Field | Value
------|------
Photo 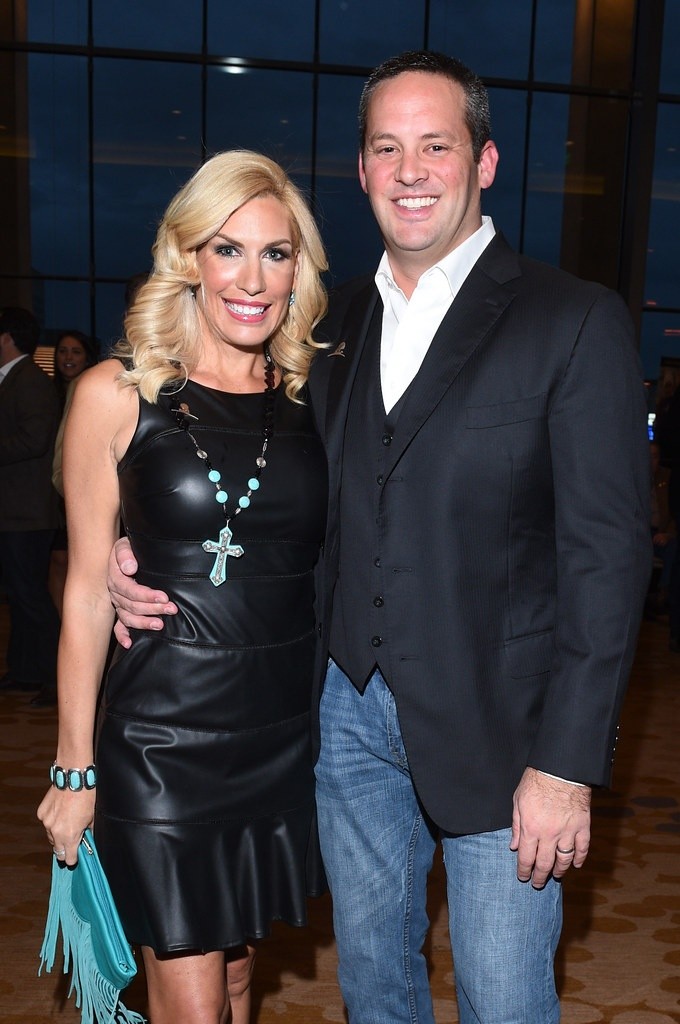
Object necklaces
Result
[167,338,275,588]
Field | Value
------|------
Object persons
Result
[107,50,654,1024]
[36,149,332,1024]
[0,306,99,707]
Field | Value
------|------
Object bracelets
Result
[49,760,97,792]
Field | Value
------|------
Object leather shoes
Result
[0,664,56,709]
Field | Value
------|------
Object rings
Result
[557,845,574,853]
